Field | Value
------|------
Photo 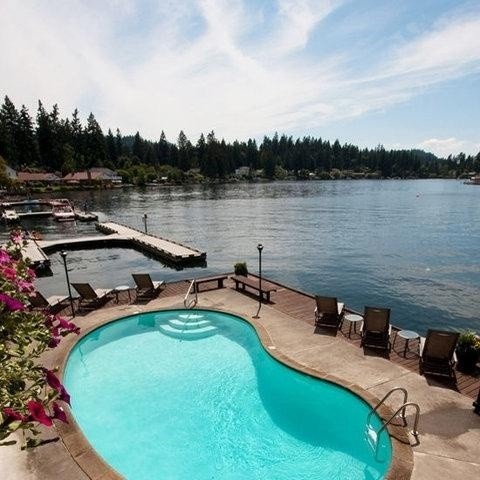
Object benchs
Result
[183,272,277,305]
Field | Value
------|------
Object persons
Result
[83,199,93,215]
[37,229,42,240]
[69,198,77,211]
[23,228,30,240]
[31,228,37,240]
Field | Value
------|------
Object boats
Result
[51,199,75,222]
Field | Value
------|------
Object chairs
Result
[312,294,461,382]
[25,273,165,309]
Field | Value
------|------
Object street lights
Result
[59,251,75,317]
[257,243,264,308]
